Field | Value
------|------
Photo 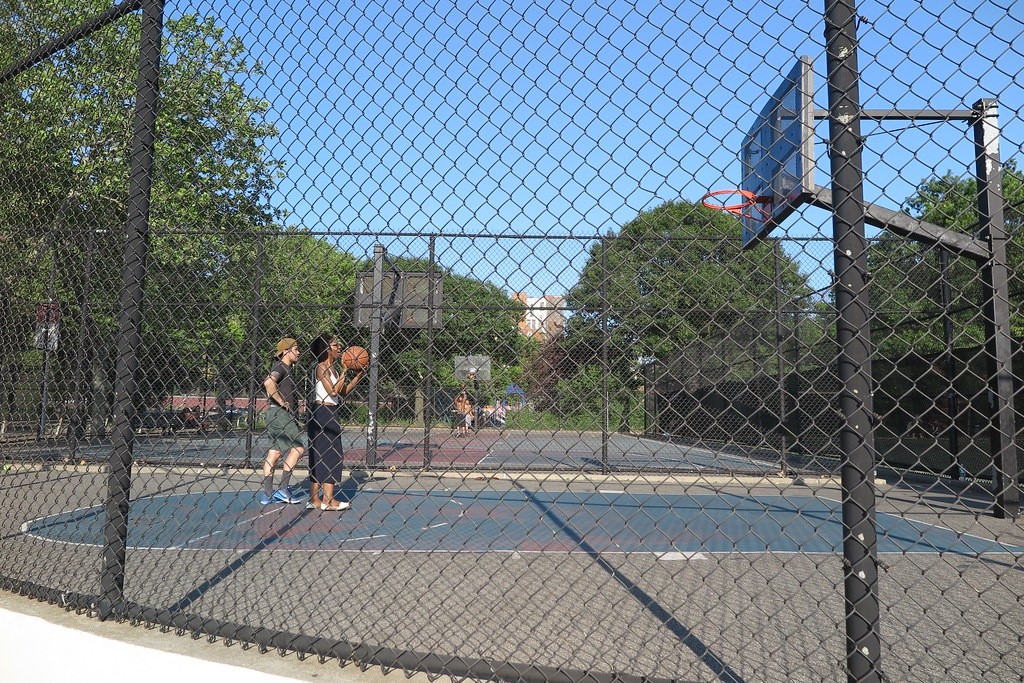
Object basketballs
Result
[344,346,368,370]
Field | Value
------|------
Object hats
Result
[274,338,298,357]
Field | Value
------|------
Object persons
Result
[306,333,369,510]
[454,394,471,437]
[178,406,207,432]
[259,338,304,504]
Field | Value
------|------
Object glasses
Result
[330,343,338,348]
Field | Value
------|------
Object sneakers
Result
[271,487,300,503]
[260,492,284,504]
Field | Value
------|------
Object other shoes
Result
[306,502,321,508]
[462,431,465,438]
[455,432,460,438]
[320,502,351,511]
